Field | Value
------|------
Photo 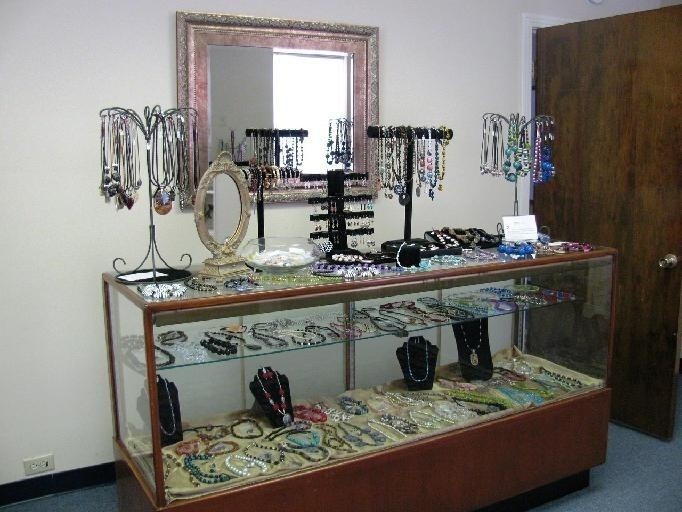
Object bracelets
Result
[244,167,301,193]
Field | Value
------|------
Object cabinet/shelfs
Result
[101,236,619,511]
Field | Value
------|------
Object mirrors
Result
[193,150,251,255]
[175,11,380,207]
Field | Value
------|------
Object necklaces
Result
[119,228,595,490]
[247,125,305,167]
[322,119,450,205]
[481,112,557,186]
[94,102,200,214]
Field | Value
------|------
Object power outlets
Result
[22,453,55,475]
